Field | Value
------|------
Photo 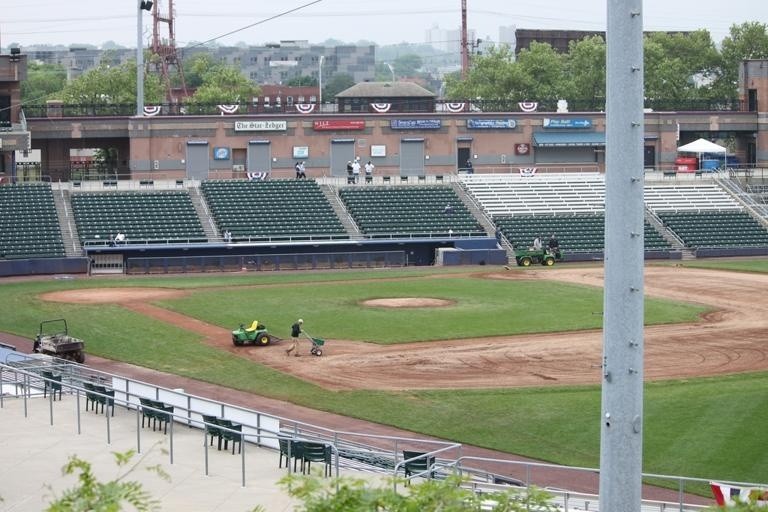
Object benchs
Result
[459,172,745,218]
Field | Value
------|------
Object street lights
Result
[439,80,448,110]
[381,60,395,82]
[317,54,324,110]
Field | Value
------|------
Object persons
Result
[495,226,501,244]
[533,234,542,249]
[444,204,453,213]
[549,234,558,248]
[108,233,114,247]
[223,229,232,242]
[115,230,126,244]
[364,161,375,182]
[300,161,306,178]
[285,319,304,357]
[352,160,361,183]
[295,162,300,178]
[465,159,474,174]
[346,161,355,184]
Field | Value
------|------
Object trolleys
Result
[299,329,325,356]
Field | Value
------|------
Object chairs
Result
[490,207,768,253]
[274,433,302,473]
[214,416,243,455]
[201,412,220,450]
[150,399,174,433]
[1,174,488,262]
[40,368,64,402]
[82,380,99,414]
[93,384,116,418]
[300,440,333,478]
[402,448,436,487]
[139,397,157,431]
